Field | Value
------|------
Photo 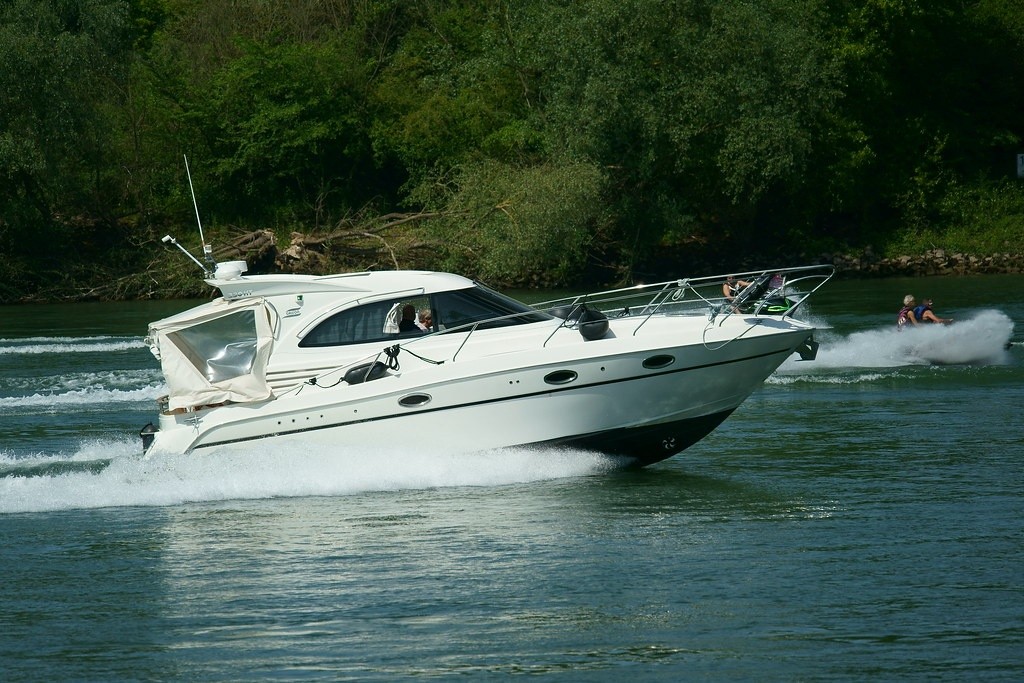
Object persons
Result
[399,305,432,334]
[723,276,750,314]
[898,294,952,334]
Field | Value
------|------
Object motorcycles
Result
[716,269,799,318]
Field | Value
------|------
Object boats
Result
[135,150,838,473]
